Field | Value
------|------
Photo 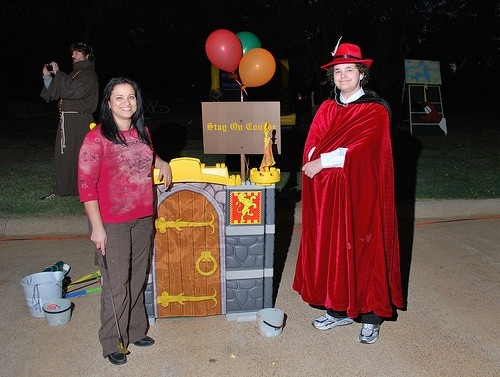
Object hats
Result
[320,35,374,71]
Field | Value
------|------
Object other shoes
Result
[41,192,59,200]
[108,351,127,365]
[133,336,155,346]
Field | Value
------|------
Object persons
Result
[41,43,97,199]
[78,78,172,365]
[292,43,403,344]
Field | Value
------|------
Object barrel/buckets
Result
[20,271,64,318]
[44,299,72,326]
[256,308,284,338]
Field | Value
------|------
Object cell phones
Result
[46,65,52,72]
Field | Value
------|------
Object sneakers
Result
[358,322,380,344]
[311,311,354,331]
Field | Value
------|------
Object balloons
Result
[205,29,242,73]
[236,32,261,55]
[239,48,276,87]
